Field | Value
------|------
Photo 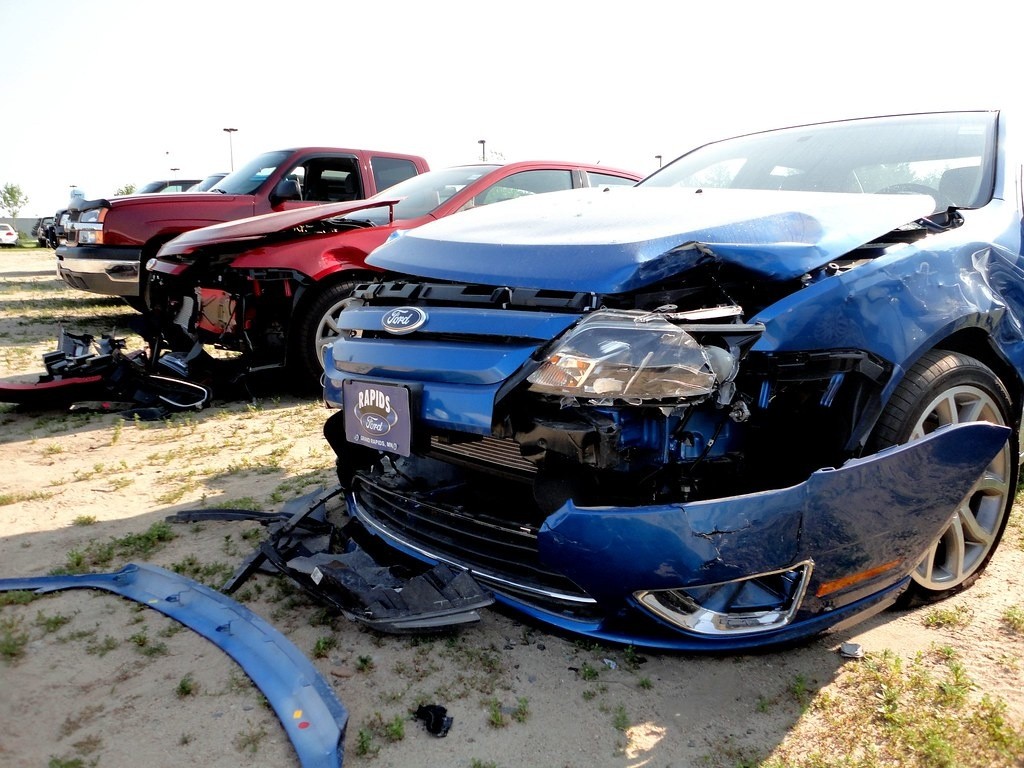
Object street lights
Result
[224,128,238,170]
[655,155,661,168]
[478,140,485,161]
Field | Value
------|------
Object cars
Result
[318,108,1024,655]
[1,224,19,248]
[38,217,54,247]
[186,173,457,205]
[145,163,646,399]
[48,180,203,250]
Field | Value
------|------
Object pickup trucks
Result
[54,147,440,313]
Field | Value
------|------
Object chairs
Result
[780,172,837,192]
[938,165,983,208]
[340,173,361,199]
